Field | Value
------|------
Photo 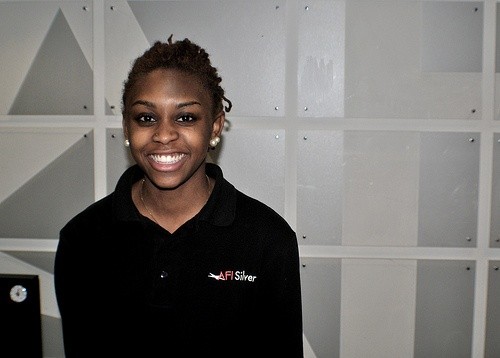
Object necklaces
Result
[139,175,212,233]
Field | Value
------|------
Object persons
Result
[53,33,304,358]
